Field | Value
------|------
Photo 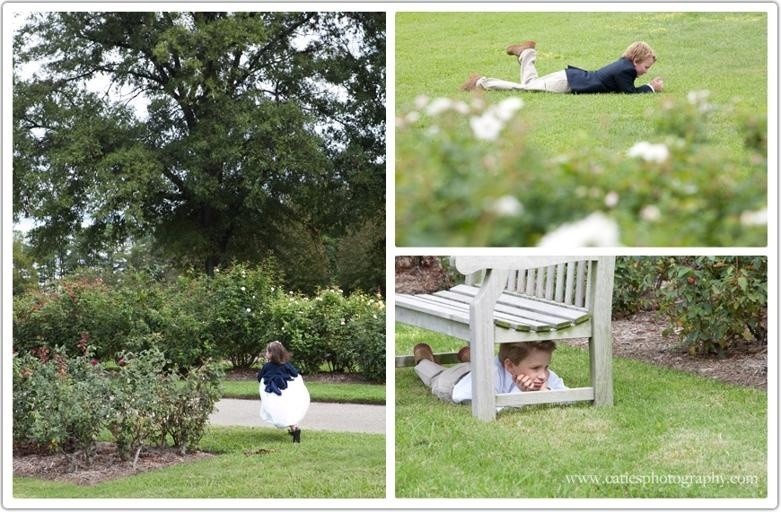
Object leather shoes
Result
[288,428,301,443]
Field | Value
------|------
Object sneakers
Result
[457,344,470,362]
[505,41,536,57]
[459,71,481,93]
[413,342,440,366]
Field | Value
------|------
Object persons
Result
[257,341,311,442]
[459,40,665,95]
[413,341,578,414]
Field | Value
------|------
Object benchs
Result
[395,254,617,423]
